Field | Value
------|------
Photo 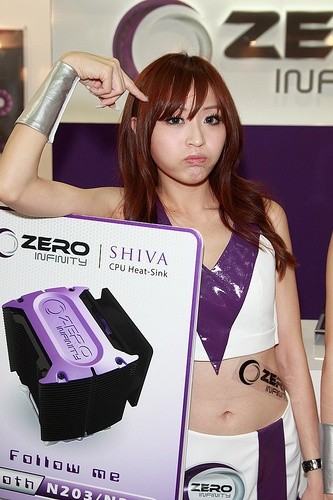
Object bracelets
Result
[302,457,323,473]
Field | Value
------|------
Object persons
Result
[322,232,333,500]
[0,50,325,500]
